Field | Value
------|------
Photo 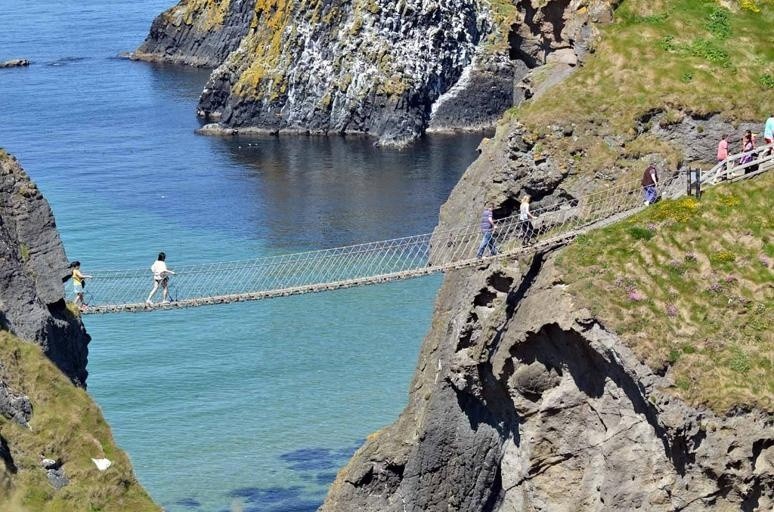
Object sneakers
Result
[644,202,649,208]
[163,300,170,304]
[147,300,153,305]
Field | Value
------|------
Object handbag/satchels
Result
[81,280,85,288]
[163,276,169,282]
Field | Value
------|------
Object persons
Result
[740,129,758,174]
[475,202,501,260]
[641,161,659,207]
[146,252,176,305]
[68,261,92,308]
[763,114,774,159]
[518,194,538,248]
[717,134,732,181]
